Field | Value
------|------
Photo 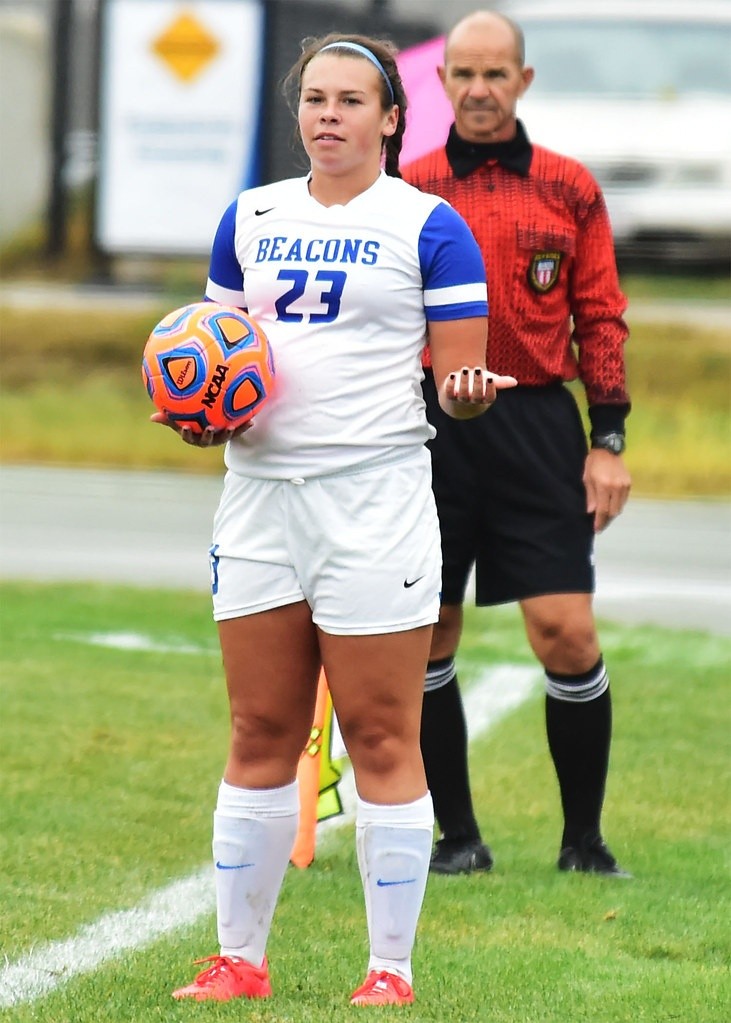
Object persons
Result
[137,34,522,1012]
[371,7,648,890]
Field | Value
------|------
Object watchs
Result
[591,431,628,458]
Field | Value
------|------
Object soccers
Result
[138,298,279,438]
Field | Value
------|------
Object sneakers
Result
[429,838,494,876]
[558,840,632,881]
[350,967,414,1008]
[171,953,271,1002]
